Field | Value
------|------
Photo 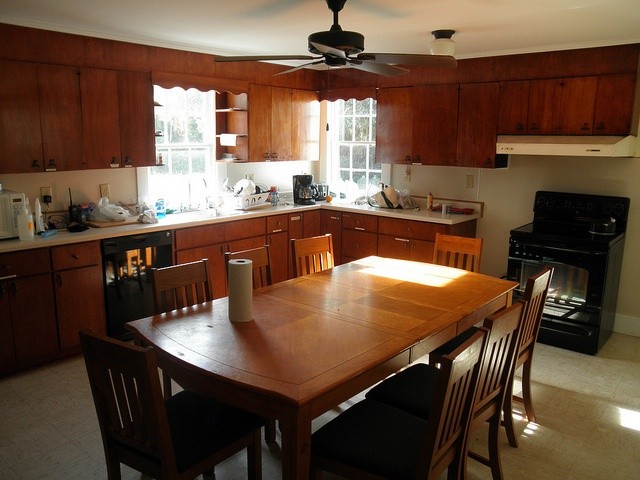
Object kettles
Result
[588,214,617,236]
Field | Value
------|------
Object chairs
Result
[291,233,335,278]
[430,231,483,272]
[150,258,215,399]
[224,244,276,445]
[310,327,491,480]
[366,300,527,480]
[429,265,554,448]
[78,328,267,479]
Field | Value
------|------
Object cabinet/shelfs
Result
[175,218,266,308]
[342,212,378,264]
[378,218,477,271]
[216,84,320,163]
[457,82,499,168]
[0,240,106,377]
[375,84,459,166]
[497,79,562,135]
[80,67,165,170]
[561,73,636,136]
[267,214,289,283]
[0,61,84,174]
[321,210,342,267]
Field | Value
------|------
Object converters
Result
[44,195,52,204]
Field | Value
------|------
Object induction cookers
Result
[512,221,623,242]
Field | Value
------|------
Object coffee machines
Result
[293,175,318,205]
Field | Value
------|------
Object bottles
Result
[270,187,278,207]
[426,192,434,211]
[18,205,34,240]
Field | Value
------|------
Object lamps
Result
[429,30,456,56]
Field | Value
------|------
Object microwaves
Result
[0,189,25,240]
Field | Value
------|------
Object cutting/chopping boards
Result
[81,216,143,227]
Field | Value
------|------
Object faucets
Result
[188,177,210,209]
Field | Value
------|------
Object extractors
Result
[495,135,640,159]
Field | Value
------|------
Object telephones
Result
[67,186,82,223]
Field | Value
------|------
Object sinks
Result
[186,207,248,220]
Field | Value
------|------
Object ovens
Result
[505,238,625,355]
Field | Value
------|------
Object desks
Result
[122,254,519,480]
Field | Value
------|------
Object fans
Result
[213,0,457,77]
